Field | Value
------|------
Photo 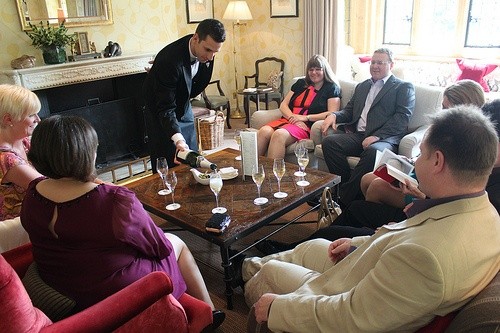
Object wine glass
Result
[163,169,181,211]
[234,128,242,161]
[272,158,289,198]
[209,170,227,213]
[251,163,268,206]
[293,140,310,186]
[156,156,172,195]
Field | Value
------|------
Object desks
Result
[237,87,275,128]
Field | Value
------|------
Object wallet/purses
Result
[205,213,231,235]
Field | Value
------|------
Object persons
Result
[20,114,224,329]
[222,103,499,333]
[0,83,117,219]
[256,79,500,255]
[142,18,228,174]
[321,48,416,206]
[256,54,345,160]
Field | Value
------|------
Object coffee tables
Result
[124,148,341,311]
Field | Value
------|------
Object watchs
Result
[306,114,309,122]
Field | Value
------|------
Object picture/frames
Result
[270,0,298,18]
[185,0,215,24]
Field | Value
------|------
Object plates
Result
[218,172,239,180]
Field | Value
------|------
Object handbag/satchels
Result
[318,187,342,229]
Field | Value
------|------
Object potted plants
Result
[23,19,76,65]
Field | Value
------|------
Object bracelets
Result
[374,135,379,141]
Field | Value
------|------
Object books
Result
[373,148,419,189]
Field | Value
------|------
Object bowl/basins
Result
[189,168,211,186]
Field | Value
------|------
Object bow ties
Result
[190,60,199,65]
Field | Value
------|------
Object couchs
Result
[0,241,213,333]
[250,77,447,175]
[247,270,500,333]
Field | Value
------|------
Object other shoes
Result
[227,249,245,291]
[254,239,289,256]
[199,310,225,333]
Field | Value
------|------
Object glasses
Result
[369,61,391,65]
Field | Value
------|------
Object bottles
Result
[176,148,218,171]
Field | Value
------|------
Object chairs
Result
[190,80,232,129]
[245,56,284,124]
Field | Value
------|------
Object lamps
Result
[222,1,253,118]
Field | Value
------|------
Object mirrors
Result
[15,0,114,32]
